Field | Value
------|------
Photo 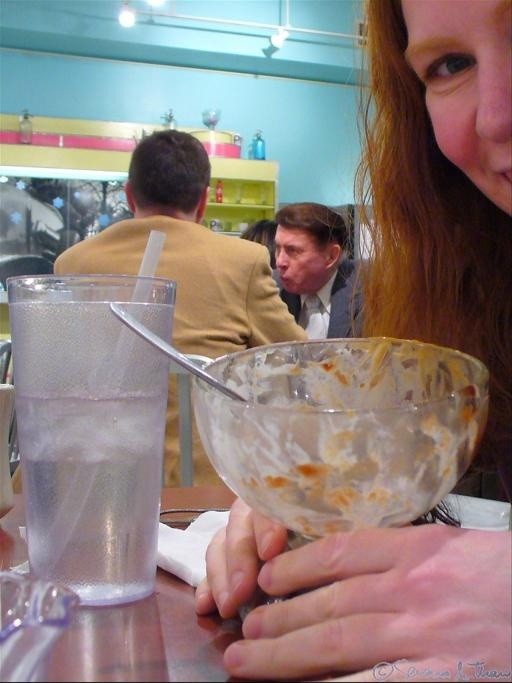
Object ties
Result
[304,295,326,339]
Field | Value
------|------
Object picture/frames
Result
[1,163,142,306]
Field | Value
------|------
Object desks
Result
[2,479,367,681]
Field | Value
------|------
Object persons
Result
[196,0,511,682]
[52,130,311,486]
[23,175,95,245]
[239,221,279,269]
[271,203,376,339]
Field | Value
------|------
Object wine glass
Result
[202,108,222,131]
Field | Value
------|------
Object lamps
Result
[117,0,293,49]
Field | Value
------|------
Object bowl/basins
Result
[192,338,492,619]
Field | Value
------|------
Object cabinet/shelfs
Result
[199,156,281,238]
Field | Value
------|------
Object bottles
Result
[18,110,36,144]
[251,128,268,160]
[215,179,223,202]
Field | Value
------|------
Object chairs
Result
[0,336,21,476]
[169,352,215,489]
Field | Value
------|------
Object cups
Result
[1,272,180,607]
[210,219,223,231]
[238,223,249,232]
